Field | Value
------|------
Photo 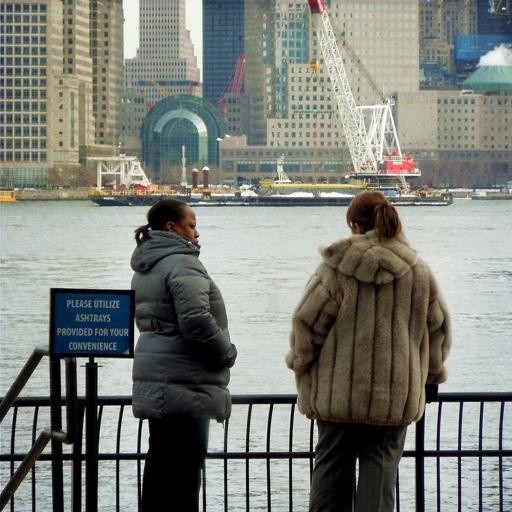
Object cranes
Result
[307,1,423,190]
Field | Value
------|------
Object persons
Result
[284,188,452,511]
[130,196,239,512]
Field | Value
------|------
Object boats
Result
[89,192,453,207]
